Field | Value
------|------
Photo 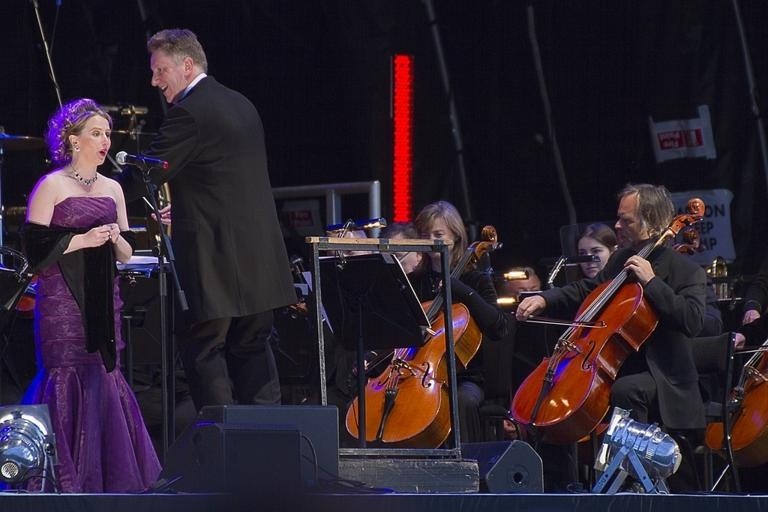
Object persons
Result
[512,180,709,484]
[741,258,766,326]
[328,203,628,450]
[128,28,293,411]
[726,318,768,352]
[25,96,166,494]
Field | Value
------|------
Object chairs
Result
[589,272,745,494]
[447,311,523,463]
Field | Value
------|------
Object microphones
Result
[116,151,169,170]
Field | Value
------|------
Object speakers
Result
[156,405,340,495]
[460,440,546,493]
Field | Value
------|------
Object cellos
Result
[345,225,499,448]
[707,339,768,466]
[511,198,705,445]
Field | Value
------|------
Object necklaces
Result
[71,166,98,186]
